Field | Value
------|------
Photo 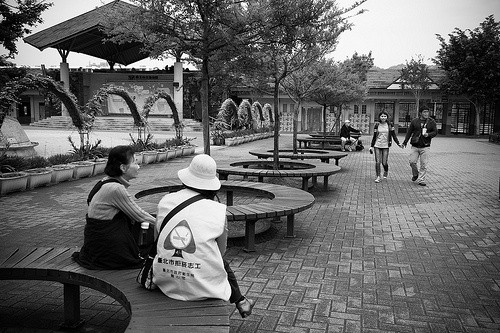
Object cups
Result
[370,149,373,154]
[422,128,427,136]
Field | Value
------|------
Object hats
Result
[177,154,221,190]
[345,120,350,123]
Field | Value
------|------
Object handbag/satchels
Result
[136,252,158,289]
[411,137,419,143]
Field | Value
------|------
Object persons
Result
[340,120,363,152]
[369,111,404,183]
[401,105,437,186]
[84,146,157,270]
[152,154,256,318]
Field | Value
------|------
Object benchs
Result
[0,131,361,333]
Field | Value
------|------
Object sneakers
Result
[375,176,382,183]
[236,297,255,318]
[383,175,388,179]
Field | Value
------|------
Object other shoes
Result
[412,174,418,181]
[419,180,426,186]
[348,147,352,151]
[341,148,345,151]
